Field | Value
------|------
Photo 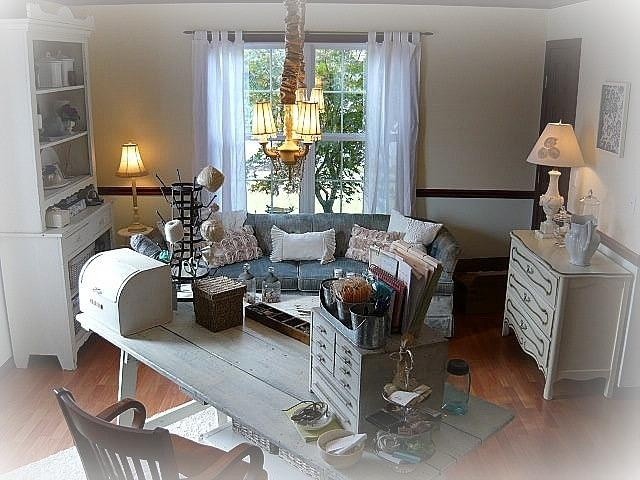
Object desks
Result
[75,302,516,479]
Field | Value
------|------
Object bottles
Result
[239,264,257,304]
[553,205,570,248]
[579,189,600,223]
[443,358,470,416]
[261,267,281,303]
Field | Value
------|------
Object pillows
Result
[215,225,262,266]
[269,225,336,264]
[387,210,444,253]
[344,224,404,265]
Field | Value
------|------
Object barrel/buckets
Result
[335,294,372,328]
[349,305,386,349]
[322,278,341,315]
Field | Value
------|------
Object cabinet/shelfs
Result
[0,2,100,233]
[308,306,450,440]
[501,228,634,400]
[0,197,113,372]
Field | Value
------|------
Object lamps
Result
[526,119,585,241]
[252,1,325,184]
[116,139,150,232]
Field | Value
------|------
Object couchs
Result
[208,213,463,338]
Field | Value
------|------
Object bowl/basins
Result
[317,429,365,467]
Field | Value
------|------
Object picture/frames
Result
[594,83,630,158]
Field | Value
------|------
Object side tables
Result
[116,226,154,249]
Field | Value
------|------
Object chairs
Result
[53,386,268,479]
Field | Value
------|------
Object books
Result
[364,240,441,335]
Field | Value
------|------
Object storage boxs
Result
[192,276,247,333]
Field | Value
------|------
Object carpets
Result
[0,398,313,479]
